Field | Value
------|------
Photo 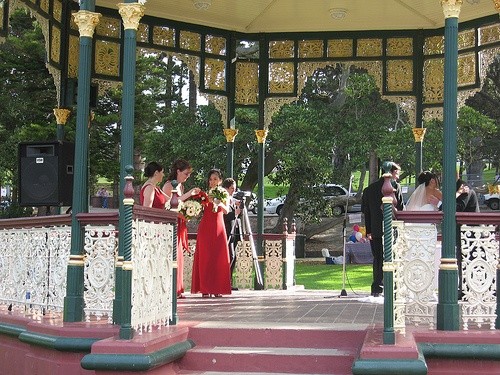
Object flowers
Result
[165,184,228,222]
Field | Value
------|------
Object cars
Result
[266,183,351,217]
[482,193,500,210]
[243,191,259,214]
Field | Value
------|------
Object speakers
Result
[17,141,75,207]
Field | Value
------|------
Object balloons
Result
[348,224,367,242]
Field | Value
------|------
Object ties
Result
[230,198,233,205]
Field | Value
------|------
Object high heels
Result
[202,294,209,297]
[210,293,222,297]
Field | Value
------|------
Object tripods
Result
[227,200,264,289]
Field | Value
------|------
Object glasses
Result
[182,171,190,177]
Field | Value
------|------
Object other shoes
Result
[231,286,238,290]
[177,294,185,299]
[371,286,384,297]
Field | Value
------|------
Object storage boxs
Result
[346,243,374,264]
[325,257,335,264]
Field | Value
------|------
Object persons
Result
[426,171,487,301]
[403,170,469,305]
[98,186,109,209]
[222,178,240,290]
[162,160,201,298]
[191,169,232,298]
[361,161,406,297]
[140,161,170,210]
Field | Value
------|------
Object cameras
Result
[233,191,256,202]
